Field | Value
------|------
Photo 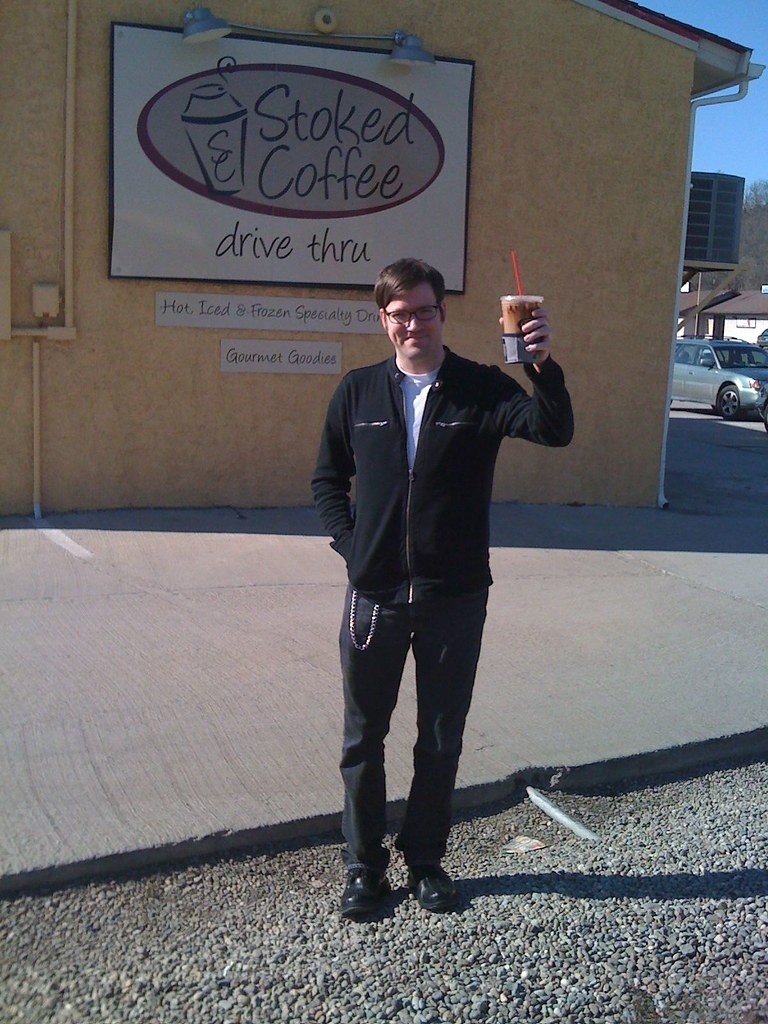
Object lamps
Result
[178,3,438,68]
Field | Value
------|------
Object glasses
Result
[383,299,441,325]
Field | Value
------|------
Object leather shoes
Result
[340,869,389,916]
[407,866,458,911]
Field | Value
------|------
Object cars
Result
[757,328,768,348]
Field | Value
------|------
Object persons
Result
[309,260,575,919]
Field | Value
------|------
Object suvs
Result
[668,333,768,420]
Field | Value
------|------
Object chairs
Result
[676,351,689,363]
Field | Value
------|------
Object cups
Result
[500,294,545,364]
